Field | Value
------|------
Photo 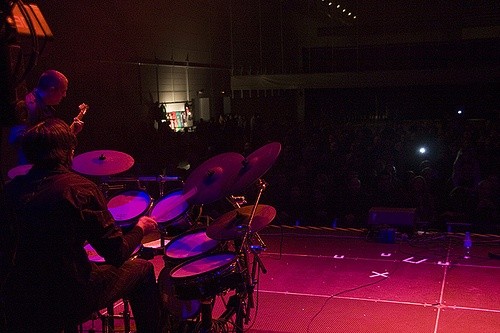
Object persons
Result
[133,112,500,234]
[0,120,172,333]
[13,70,85,166]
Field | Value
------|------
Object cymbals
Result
[205,204,277,241]
[182,152,244,204]
[71,150,135,175]
[221,142,281,194]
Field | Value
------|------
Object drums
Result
[164,226,228,271]
[106,188,153,232]
[169,250,237,300]
[147,188,203,240]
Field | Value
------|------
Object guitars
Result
[70,103,88,134]
